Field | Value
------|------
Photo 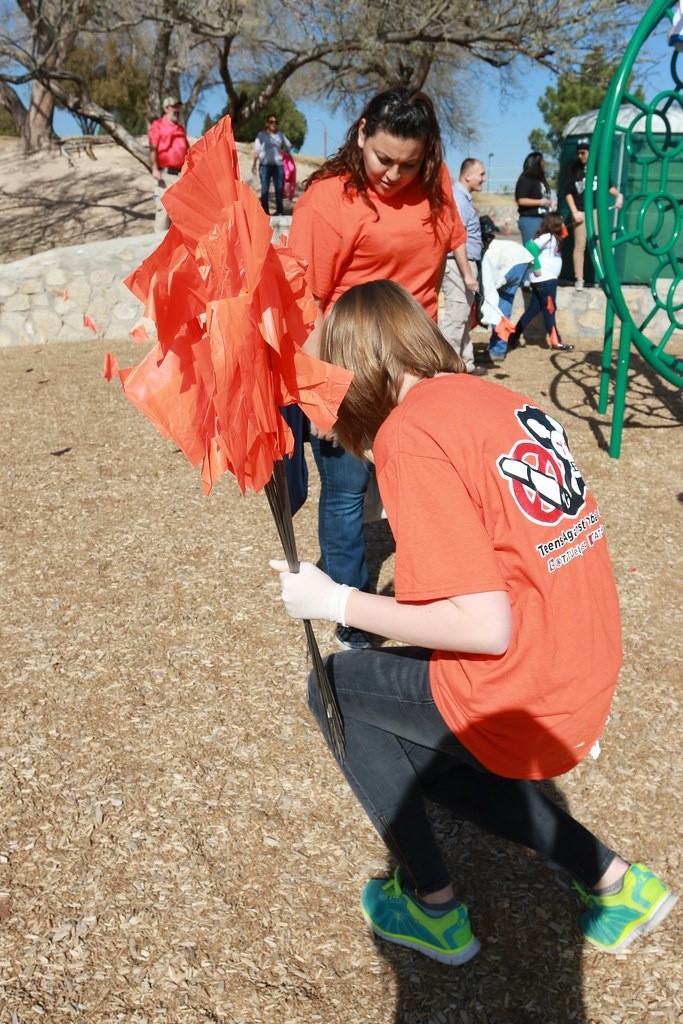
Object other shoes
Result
[553,342,574,353]
[334,623,372,652]
[575,280,585,290]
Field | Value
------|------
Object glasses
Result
[269,120,278,124]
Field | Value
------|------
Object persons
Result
[474,214,574,363]
[441,158,487,375]
[564,141,623,292]
[514,151,551,291]
[269,279,680,968]
[251,114,292,215]
[286,89,466,653]
[148,96,190,232]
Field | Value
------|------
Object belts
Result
[160,167,183,176]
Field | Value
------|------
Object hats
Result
[162,96,183,108]
[576,135,591,150]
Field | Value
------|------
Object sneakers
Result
[570,862,680,954]
[360,864,482,967]
